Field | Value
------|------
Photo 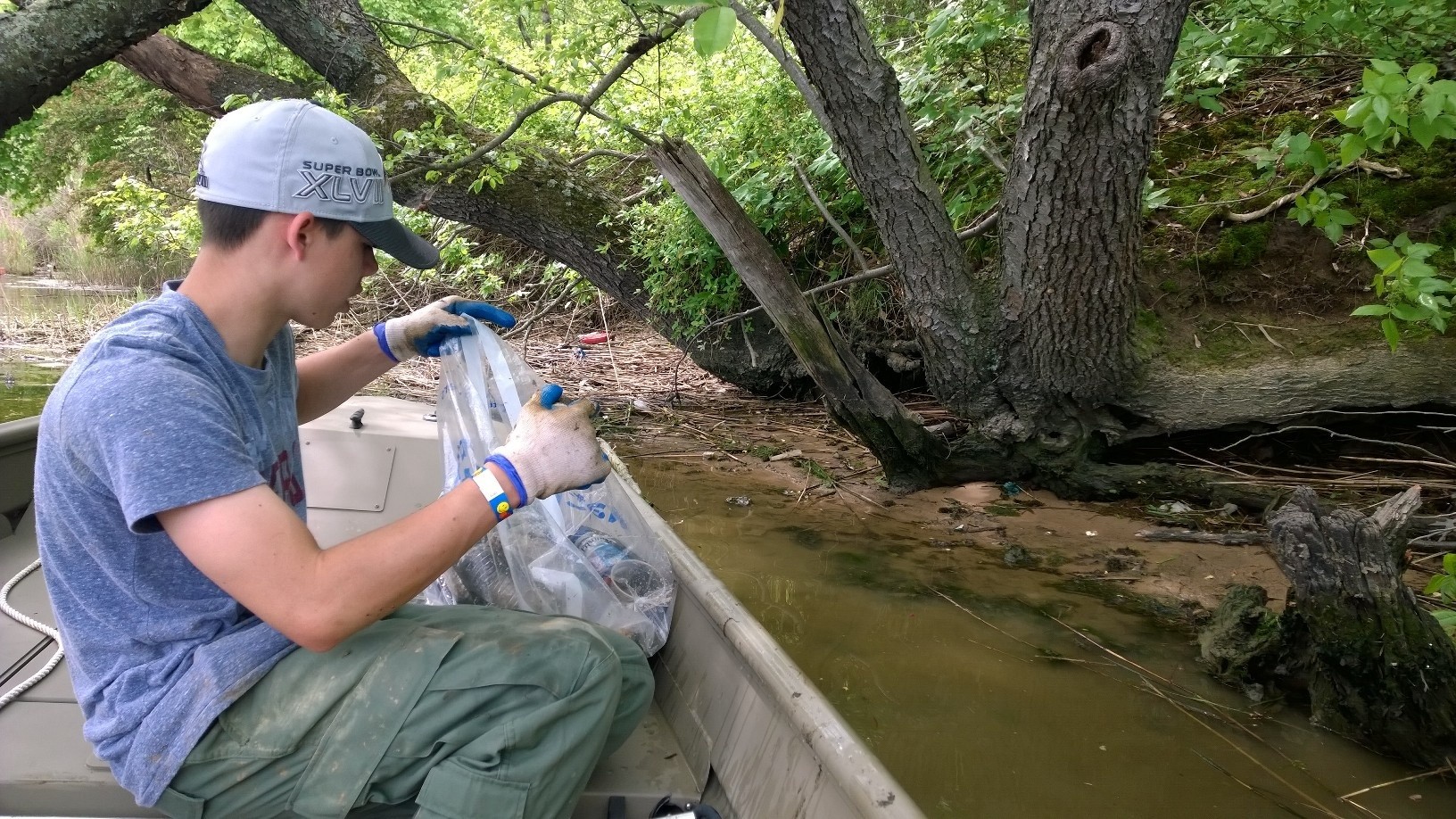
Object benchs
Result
[0,701,713,817]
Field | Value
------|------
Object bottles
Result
[565,526,652,601]
[454,544,526,611]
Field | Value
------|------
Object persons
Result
[33,99,653,818]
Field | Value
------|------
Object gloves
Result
[373,295,516,364]
[483,384,612,510]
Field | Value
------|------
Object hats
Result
[192,97,440,270]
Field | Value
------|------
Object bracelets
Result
[468,468,515,522]
